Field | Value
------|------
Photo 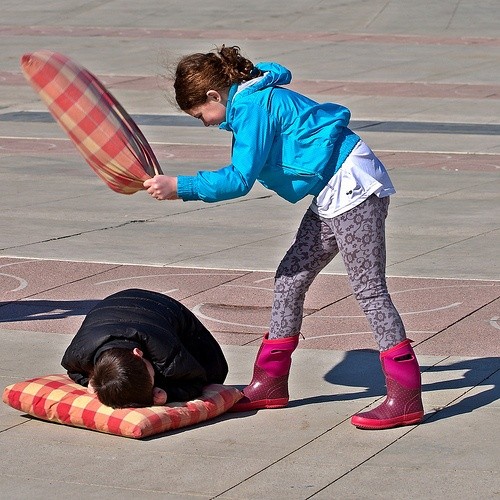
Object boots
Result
[217,332,298,413]
[350,338,426,432]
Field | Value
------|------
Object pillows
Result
[2,375,244,438]
[21,50,163,195]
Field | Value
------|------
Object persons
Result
[142,43,426,430]
[61,289,233,408]
